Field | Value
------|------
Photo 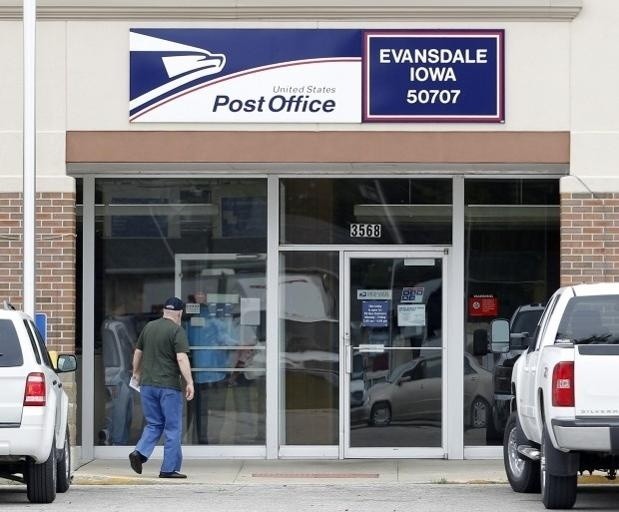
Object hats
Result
[163,297,184,310]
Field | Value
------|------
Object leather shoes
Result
[129,450,142,474]
[159,472,187,478]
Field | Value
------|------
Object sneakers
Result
[98,429,119,446]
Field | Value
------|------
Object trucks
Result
[140,274,367,425]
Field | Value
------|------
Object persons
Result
[98,294,133,446]
[185,288,266,444]
[128,298,196,478]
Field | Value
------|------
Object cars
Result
[0,311,77,503]
[364,302,550,445]
[103,321,136,446]
[503,282,619,508]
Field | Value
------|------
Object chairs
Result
[573,309,604,340]
